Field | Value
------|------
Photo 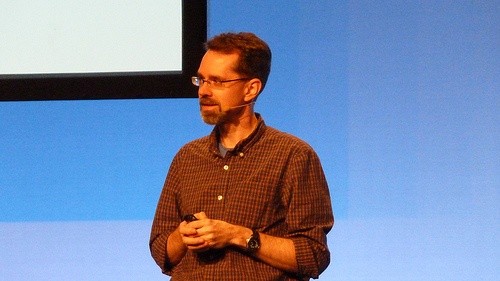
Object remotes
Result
[184,214,198,224]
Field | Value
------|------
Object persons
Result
[148,31,334,281]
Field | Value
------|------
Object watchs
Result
[245,228,261,255]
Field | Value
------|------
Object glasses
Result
[191,76,252,88]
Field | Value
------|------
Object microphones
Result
[222,102,255,112]
